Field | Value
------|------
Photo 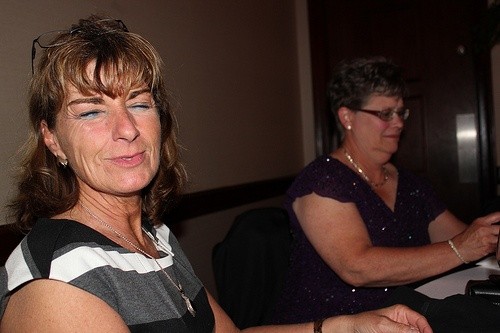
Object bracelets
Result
[312,315,325,333]
[443,237,471,267]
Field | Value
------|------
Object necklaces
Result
[74,194,198,319]
[338,142,389,187]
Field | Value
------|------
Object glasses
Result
[349,107,409,121]
[31,18,128,73]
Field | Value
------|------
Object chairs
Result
[212,206,289,330]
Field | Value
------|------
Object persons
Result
[286,58,499,313]
[0,13,440,333]
